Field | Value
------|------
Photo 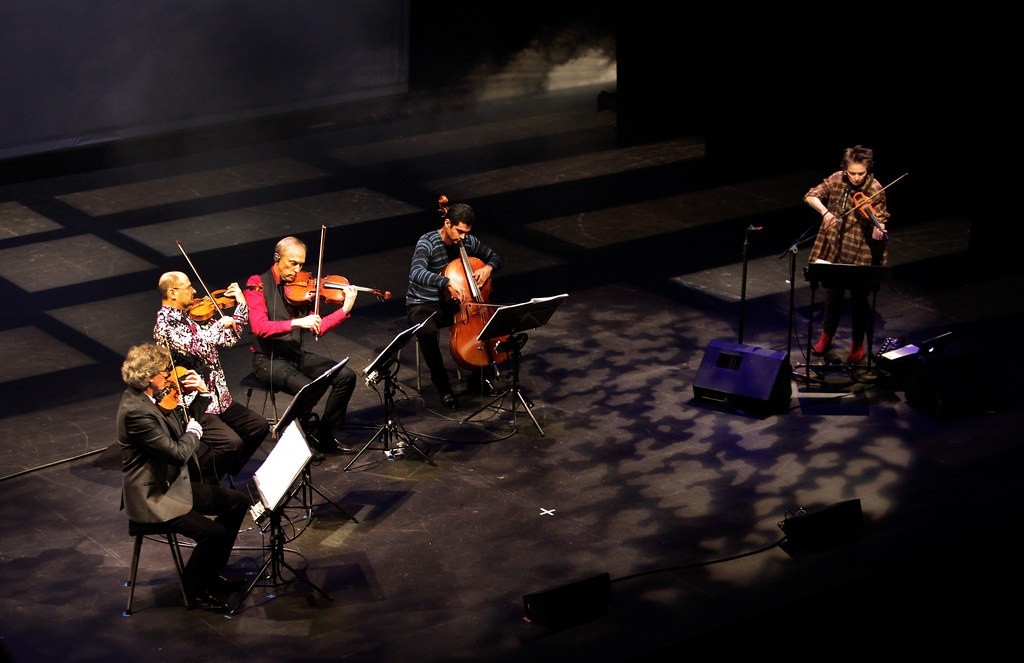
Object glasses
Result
[173,285,193,293]
[154,369,171,379]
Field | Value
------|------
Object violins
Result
[155,362,221,415]
[283,271,393,308]
[187,282,266,323]
[851,191,890,242]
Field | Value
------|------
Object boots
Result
[847,340,864,362]
[812,330,832,353]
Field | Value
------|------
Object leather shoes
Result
[311,447,324,459]
[441,393,459,409]
[313,436,354,453]
[476,388,502,396]
[190,588,240,610]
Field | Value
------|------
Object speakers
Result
[693,339,792,416]
[522,571,613,631]
[777,499,867,560]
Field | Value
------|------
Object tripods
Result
[231,323,436,616]
[459,294,570,435]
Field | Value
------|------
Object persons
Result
[405,204,500,409]
[244,236,358,460]
[116,344,249,614]
[153,271,270,485]
[803,145,891,363]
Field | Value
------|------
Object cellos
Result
[437,193,516,397]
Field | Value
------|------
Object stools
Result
[125,522,192,615]
[240,371,279,417]
[392,316,462,393]
